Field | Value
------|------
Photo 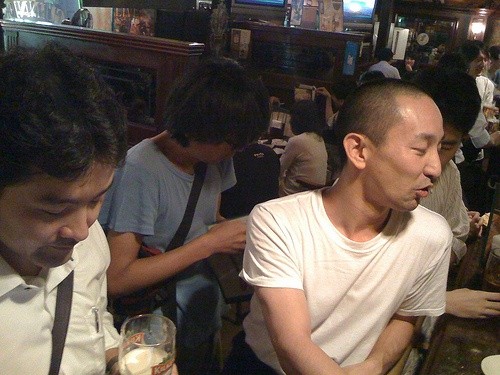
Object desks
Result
[417,206,500,375]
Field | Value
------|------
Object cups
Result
[485,248,500,292]
[482,98,496,119]
[117,313,176,374]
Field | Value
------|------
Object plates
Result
[481,355,500,375]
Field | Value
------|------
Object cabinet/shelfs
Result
[0,20,373,146]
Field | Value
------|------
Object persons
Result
[240,78,453,375]
[98,60,251,375]
[0,40,181,375]
[222,42,500,319]
